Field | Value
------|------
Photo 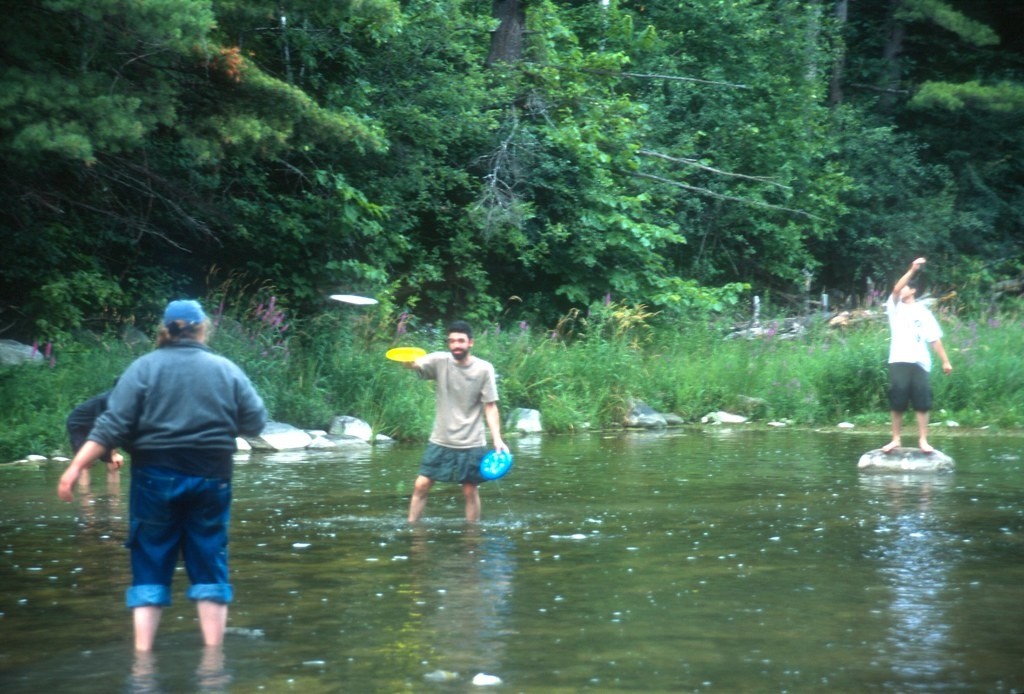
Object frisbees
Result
[478,450,513,481]
[384,346,425,361]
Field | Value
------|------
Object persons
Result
[402,321,509,523]
[881,257,953,453]
[58,299,268,650]
[67,378,123,488]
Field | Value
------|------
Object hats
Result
[162,298,204,327]
